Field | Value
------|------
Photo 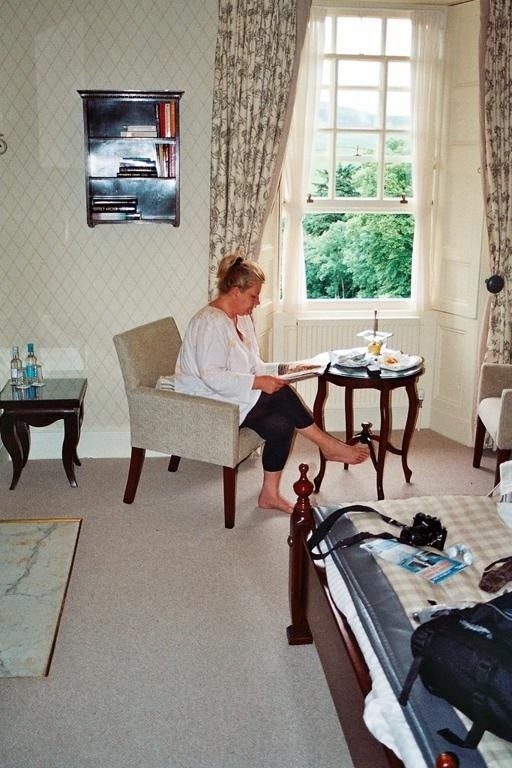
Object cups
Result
[17,367,30,389]
[30,365,44,387]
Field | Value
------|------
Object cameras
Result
[399,511,448,551]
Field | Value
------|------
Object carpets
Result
[0,519,82,677]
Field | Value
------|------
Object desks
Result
[310,348,424,500]
[0,378,87,491]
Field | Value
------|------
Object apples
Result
[367,343,381,355]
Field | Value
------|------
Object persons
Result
[171,255,371,515]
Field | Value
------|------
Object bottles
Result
[448,543,461,558]
[460,544,473,564]
[25,344,37,384]
[11,346,22,385]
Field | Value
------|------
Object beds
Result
[287,465,512,766]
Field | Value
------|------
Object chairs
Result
[113,317,297,530]
[473,363,511,486]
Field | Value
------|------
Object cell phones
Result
[366,364,382,375]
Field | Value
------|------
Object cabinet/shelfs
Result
[76,90,184,227]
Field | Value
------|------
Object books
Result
[278,360,331,384]
[90,194,142,221]
[121,100,176,138]
[117,143,177,178]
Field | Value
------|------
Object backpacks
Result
[411,590,510,746]
